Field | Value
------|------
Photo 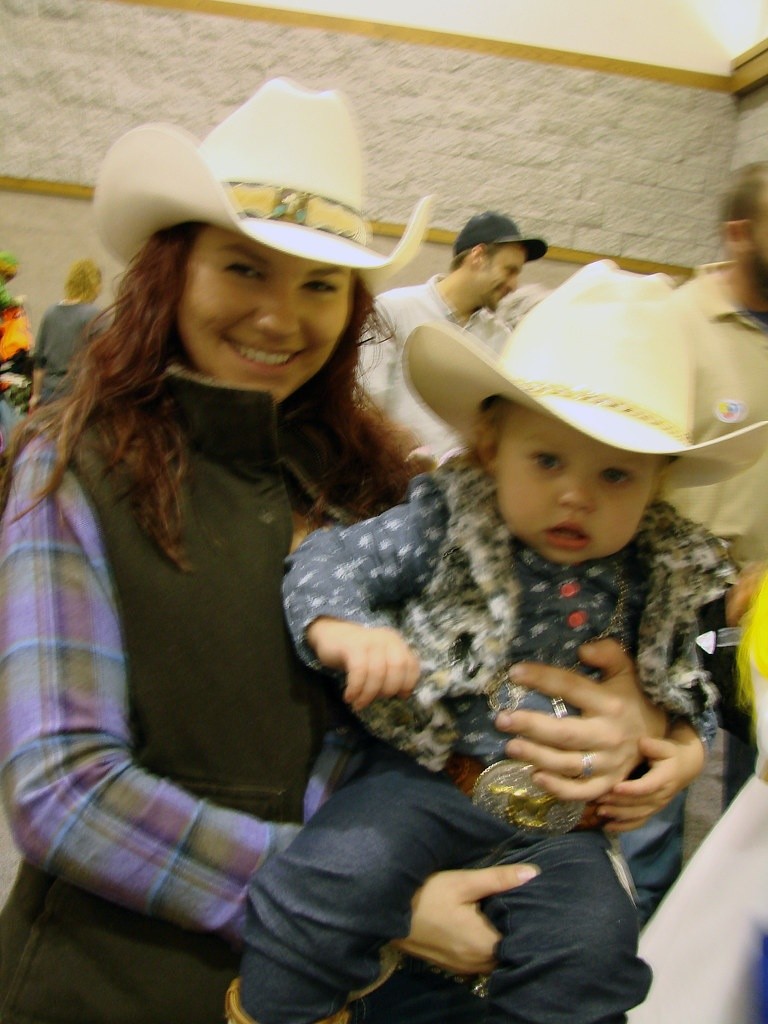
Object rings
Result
[582,753,592,776]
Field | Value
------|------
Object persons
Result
[0,79,768,1024]
[225,261,768,1024]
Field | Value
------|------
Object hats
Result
[399,258,768,457]
[451,209,547,257]
[94,76,432,279]
